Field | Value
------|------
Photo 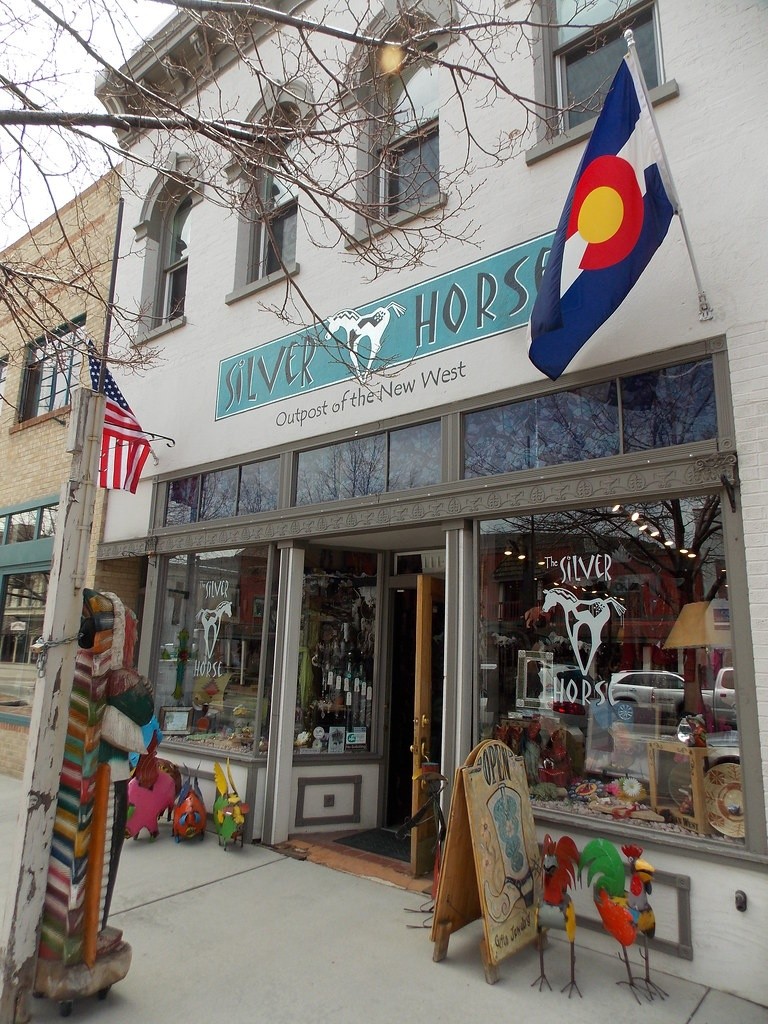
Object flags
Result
[528,50,674,381]
[88,339,150,493]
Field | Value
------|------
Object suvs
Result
[601,668,713,723]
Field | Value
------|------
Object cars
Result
[712,665,738,730]
[475,659,608,757]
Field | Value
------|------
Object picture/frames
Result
[516,649,553,710]
[159,706,194,735]
